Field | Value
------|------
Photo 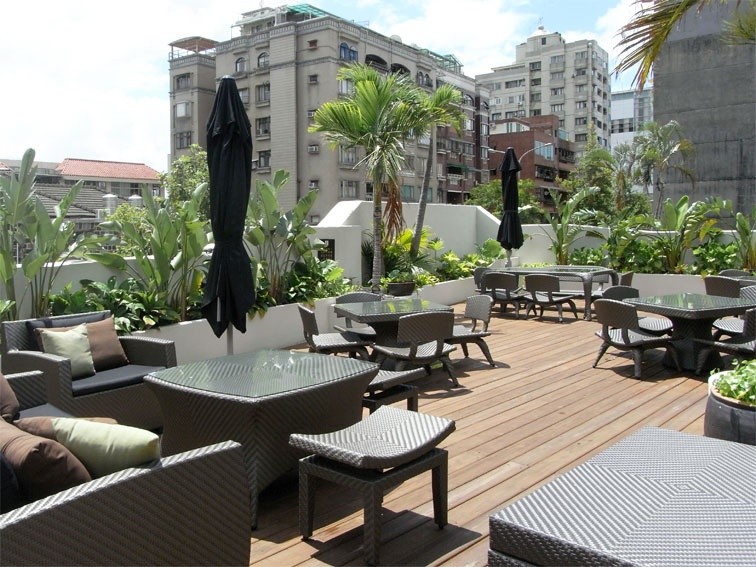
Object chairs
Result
[0,310,177,432]
[297,267,755,383]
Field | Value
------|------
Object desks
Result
[727,275,756,286]
[331,299,454,364]
[480,267,620,322]
[622,292,755,366]
[145,350,379,527]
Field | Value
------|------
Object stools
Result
[366,368,424,416]
[486,427,756,567]
[288,405,456,567]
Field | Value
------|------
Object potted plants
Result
[380,266,439,296]
[704,359,756,444]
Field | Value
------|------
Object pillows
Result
[0,371,21,422]
[35,324,96,377]
[0,418,91,487]
[13,416,160,471]
[30,315,129,372]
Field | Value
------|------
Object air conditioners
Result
[308,146,319,152]
[309,183,316,186]
[307,112,316,117]
[256,129,262,135]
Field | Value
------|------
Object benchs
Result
[0,370,251,567]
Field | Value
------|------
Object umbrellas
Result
[497,146,524,267]
[203,76,256,357]
